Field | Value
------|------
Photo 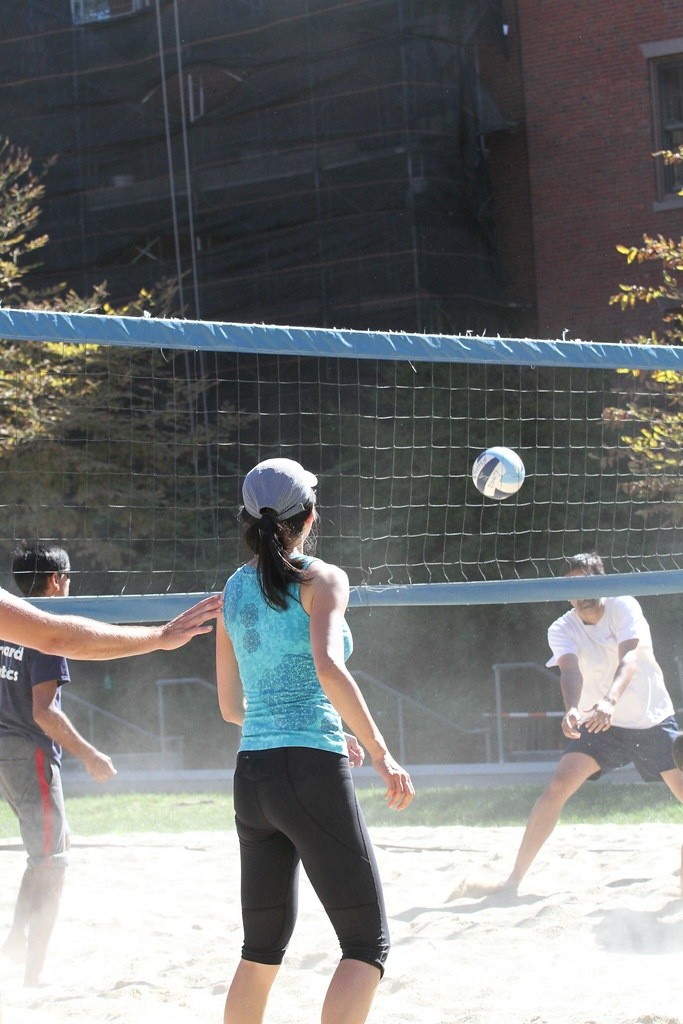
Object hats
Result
[242,456,318,523]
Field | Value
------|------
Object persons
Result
[0,586,224,661]
[505,552,683,875]
[0,545,119,985]
[215,458,416,1024]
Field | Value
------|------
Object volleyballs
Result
[471,445,526,500]
